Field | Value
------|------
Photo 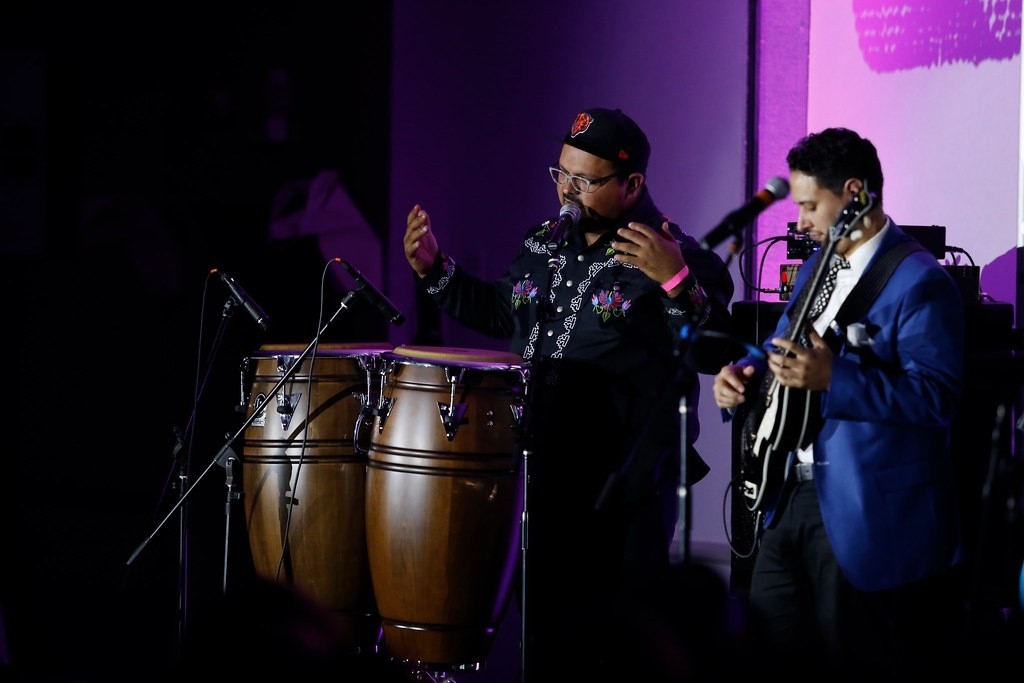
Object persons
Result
[399,107,744,683]
[712,126,967,683]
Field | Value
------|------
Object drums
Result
[242,342,395,660]
[363,343,530,665]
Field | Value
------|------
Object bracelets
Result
[666,272,699,298]
[660,264,691,291]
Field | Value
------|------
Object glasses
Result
[549,166,619,194]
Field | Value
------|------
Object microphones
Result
[701,177,789,250]
[343,260,405,327]
[546,203,584,252]
[216,270,272,331]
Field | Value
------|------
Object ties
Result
[795,256,850,350]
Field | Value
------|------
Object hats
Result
[562,107,651,174]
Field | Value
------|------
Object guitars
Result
[729,194,881,531]
